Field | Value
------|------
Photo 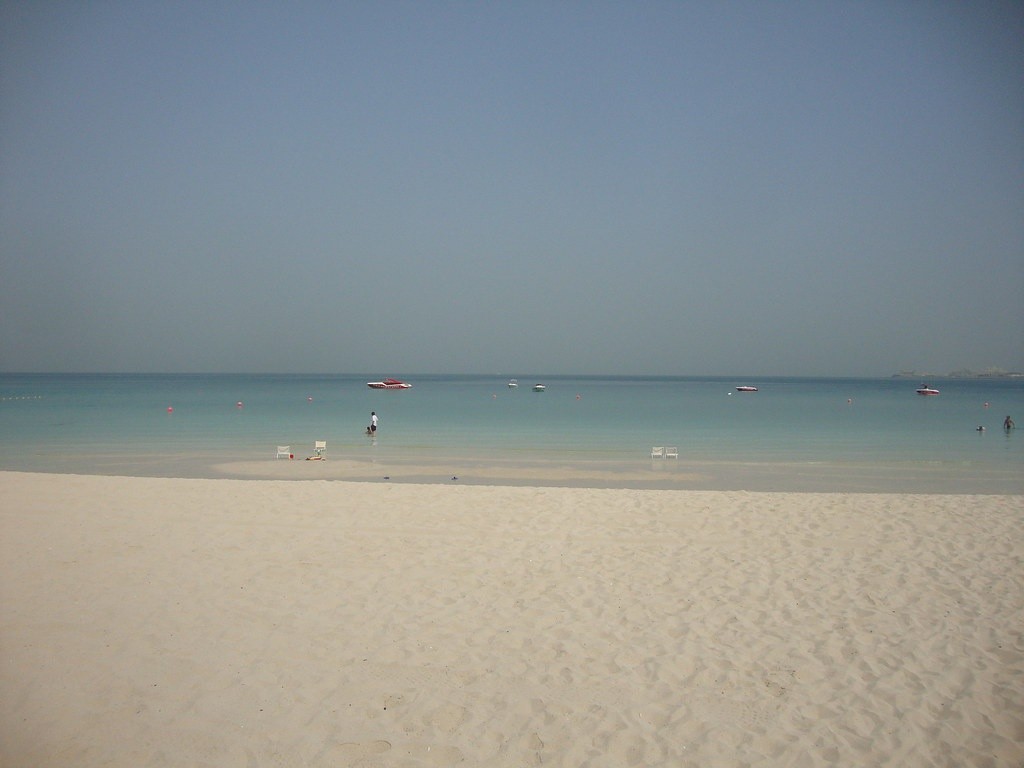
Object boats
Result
[367,378,412,389]
[736,386,758,391]
[916,388,939,394]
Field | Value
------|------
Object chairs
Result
[651,446,664,459]
[314,440,327,455]
[276,446,290,459]
[665,447,679,459]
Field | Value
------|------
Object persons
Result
[1004,416,1015,429]
[371,412,378,436]
[925,385,928,389]
[366,427,371,434]
